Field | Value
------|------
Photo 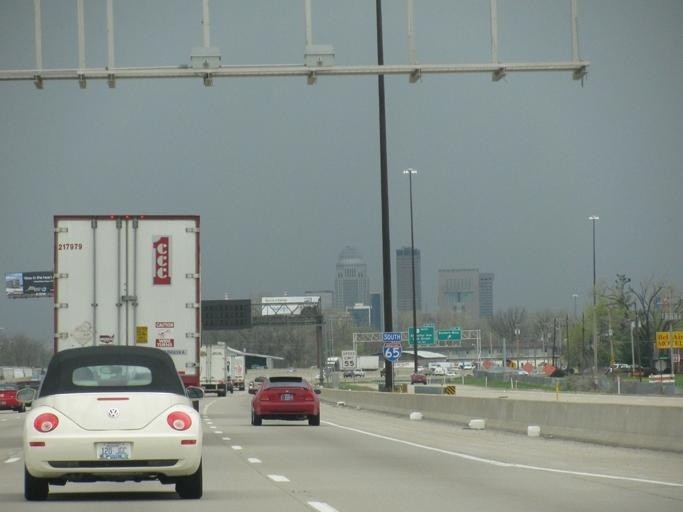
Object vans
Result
[253,377,267,393]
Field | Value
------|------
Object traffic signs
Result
[408,326,434,345]
[439,328,461,340]
[341,350,356,368]
[654,331,682,349]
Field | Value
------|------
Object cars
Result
[16,380,40,406]
[249,382,253,393]
[411,372,427,385]
[252,376,321,426]
[0,384,25,413]
[344,370,354,378]
[353,368,365,377]
[607,364,644,374]
[15,343,206,502]
[381,368,396,377]
[416,362,476,376]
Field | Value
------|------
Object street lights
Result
[513,322,522,372]
[571,293,579,316]
[587,214,600,392]
[403,166,419,375]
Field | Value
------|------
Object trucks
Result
[326,357,340,372]
[226,355,234,394]
[53,214,201,413]
[201,343,227,397]
[234,355,246,391]
